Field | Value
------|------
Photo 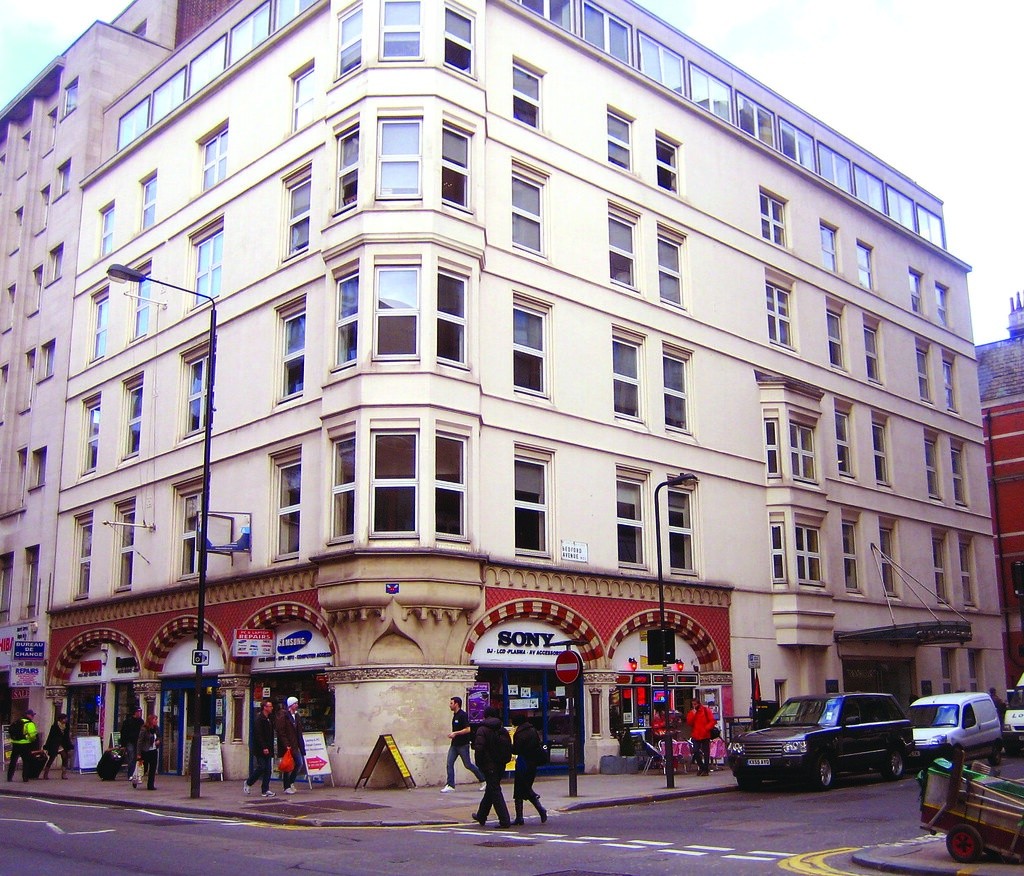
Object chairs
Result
[640,737,726,776]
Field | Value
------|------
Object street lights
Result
[108,264,218,798]
[655,473,698,789]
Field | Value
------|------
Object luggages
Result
[97,751,125,781]
[28,751,48,779]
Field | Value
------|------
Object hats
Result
[136,707,143,711]
[25,710,36,716]
[287,697,298,707]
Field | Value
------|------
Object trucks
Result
[1002,671,1024,755]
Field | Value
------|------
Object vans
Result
[906,692,1002,774]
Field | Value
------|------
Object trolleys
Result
[919,743,1024,864]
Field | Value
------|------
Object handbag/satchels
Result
[710,727,719,740]
[278,749,295,772]
[536,745,546,759]
[133,761,144,784]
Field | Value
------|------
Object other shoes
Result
[511,818,524,824]
[541,809,547,823]
[472,813,485,825]
[701,773,708,776]
[697,768,703,776]
[284,784,297,793]
[494,825,510,828]
[479,782,486,790]
[262,791,276,797]
[244,780,250,794]
[440,785,455,792]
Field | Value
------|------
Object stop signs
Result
[554,651,580,686]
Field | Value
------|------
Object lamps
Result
[675,659,685,672]
[629,657,638,672]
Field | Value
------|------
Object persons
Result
[243,699,276,797]
[120,705,145,781]
[136,714,161,791]
[989,688,1006,724]
[511,713,548,826]
[276,696,306,794]
[7,709,39,782]
[440,697,488,793]
[686,698,717,777]
[43,713,75,780]
[472,706,512,829]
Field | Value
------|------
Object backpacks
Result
[8,719,33,740]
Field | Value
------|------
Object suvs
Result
[725,692,916,792]
